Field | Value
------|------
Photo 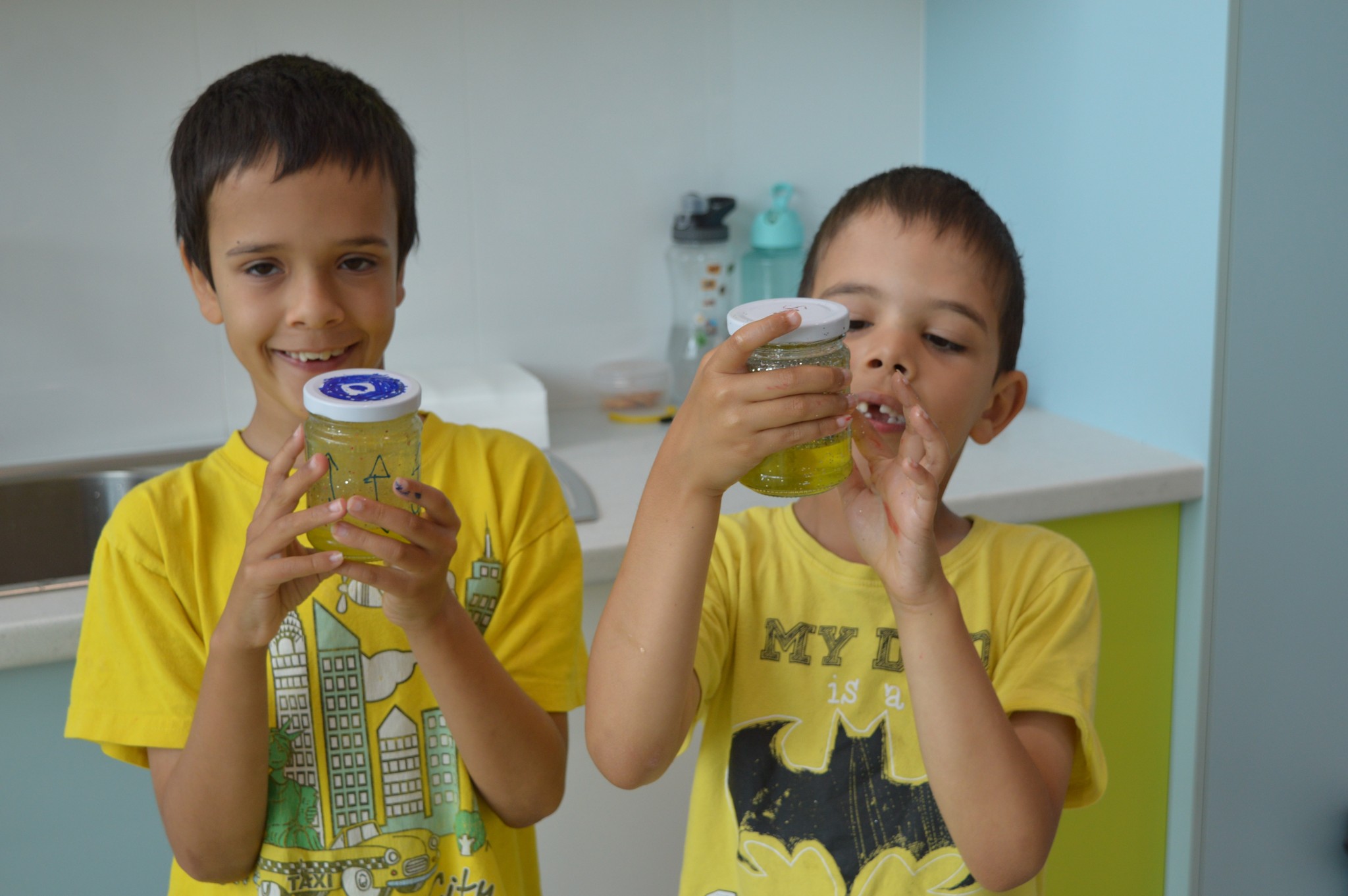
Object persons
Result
[64,54,591,896]
[584,166,1107,896]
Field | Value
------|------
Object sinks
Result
[0,468,167,593]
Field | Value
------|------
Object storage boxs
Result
[404,362,551,455]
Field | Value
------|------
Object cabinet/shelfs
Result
[0,504,1177,896]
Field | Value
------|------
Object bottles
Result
[303,368,424,562]
[738,182,810,305]
[667,195,740,410]
[727,298,853,498]
